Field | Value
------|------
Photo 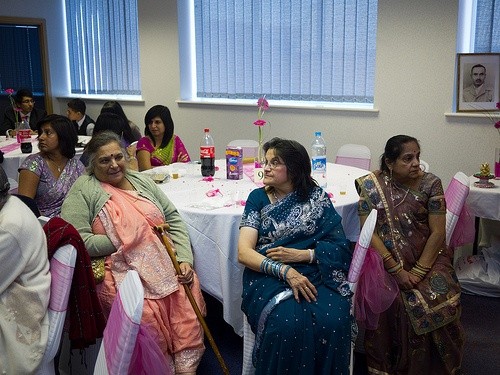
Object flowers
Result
[7,89,22,130]
[253,94,270,163]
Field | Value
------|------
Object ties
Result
[24,116,29,121]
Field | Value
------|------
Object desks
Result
[0,135,96,182]
[136,155,377,339]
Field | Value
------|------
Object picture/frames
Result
[457,53,500,112]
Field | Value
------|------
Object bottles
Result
[200,128,216,177]
[18,116,33,153]
[310,132,327,189]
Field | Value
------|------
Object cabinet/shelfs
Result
[454,172,500,297]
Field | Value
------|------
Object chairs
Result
[36,244,78,375]
[228,140,263,158]
[336,144,371,172]
[92,270,144,375]
[242,208,378,375]
[445,172,468,246]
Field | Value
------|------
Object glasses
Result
[262,161,285,168]
[22,100,34,104]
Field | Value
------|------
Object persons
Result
[238,139,354,375]
[61,132,206,375]
[136,106,190,171]
[0,88,46,136]
[19,116,87,218]
[464,63,494,103]
[67,98,140,169]
[0,165,51,375]
[354,134,463,375]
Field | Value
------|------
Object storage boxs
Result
[225,147,243,179]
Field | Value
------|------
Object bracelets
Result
[308,248,314,264]
[260,257,291,283]
[408,262,431,280]
[381,253,403,275]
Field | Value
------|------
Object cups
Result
[15,122,20,143]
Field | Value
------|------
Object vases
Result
[14,122,25,130]
[254,159,265,182]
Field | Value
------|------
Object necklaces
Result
[388,182,410,209]
[272,193,281,204]
[50,159,63,173]
